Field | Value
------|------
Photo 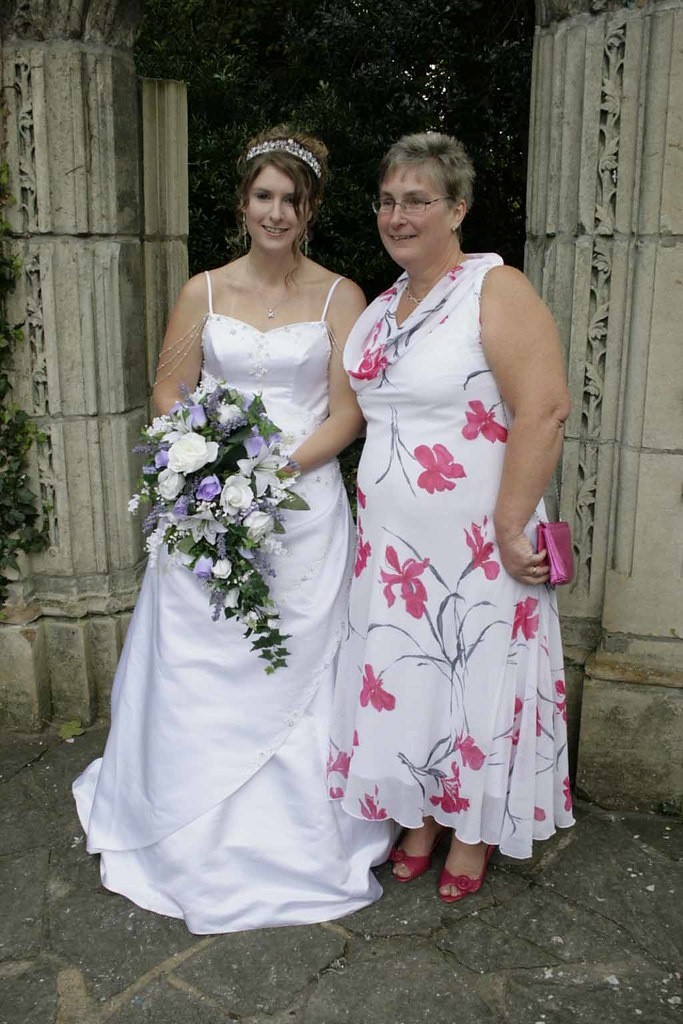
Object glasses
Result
[371,195,450,213]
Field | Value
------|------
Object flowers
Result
[123,368,310,675]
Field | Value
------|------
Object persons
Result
[72,123,400,930]
[338,133,580,897]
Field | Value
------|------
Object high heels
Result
[391,827,451,882]
[438,844,495,903]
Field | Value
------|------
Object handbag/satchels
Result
[537,521,576,586]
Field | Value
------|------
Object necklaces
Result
[403,247,466,305]
[240,256,308,322]
